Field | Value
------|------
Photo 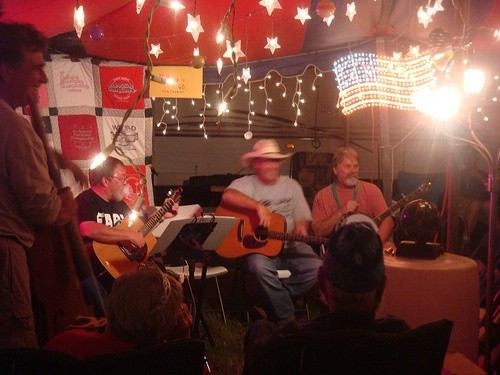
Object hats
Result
[240,139,294,170]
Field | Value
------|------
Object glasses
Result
[110,174,128,183]
[177,305,184,317]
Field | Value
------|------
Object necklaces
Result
[332,180,359,209]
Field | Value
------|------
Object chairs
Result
[152,202,309,326]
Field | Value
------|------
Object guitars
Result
[337,178,432,239]
[212,204,334,259]
[79,187,181,289]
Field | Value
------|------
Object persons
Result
[0,21,89,375]
[222,139,324,330]
[74,156,180,297]
[44,261,211,375]
[312,147,394,313]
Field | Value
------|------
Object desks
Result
[382,250,478,365]
